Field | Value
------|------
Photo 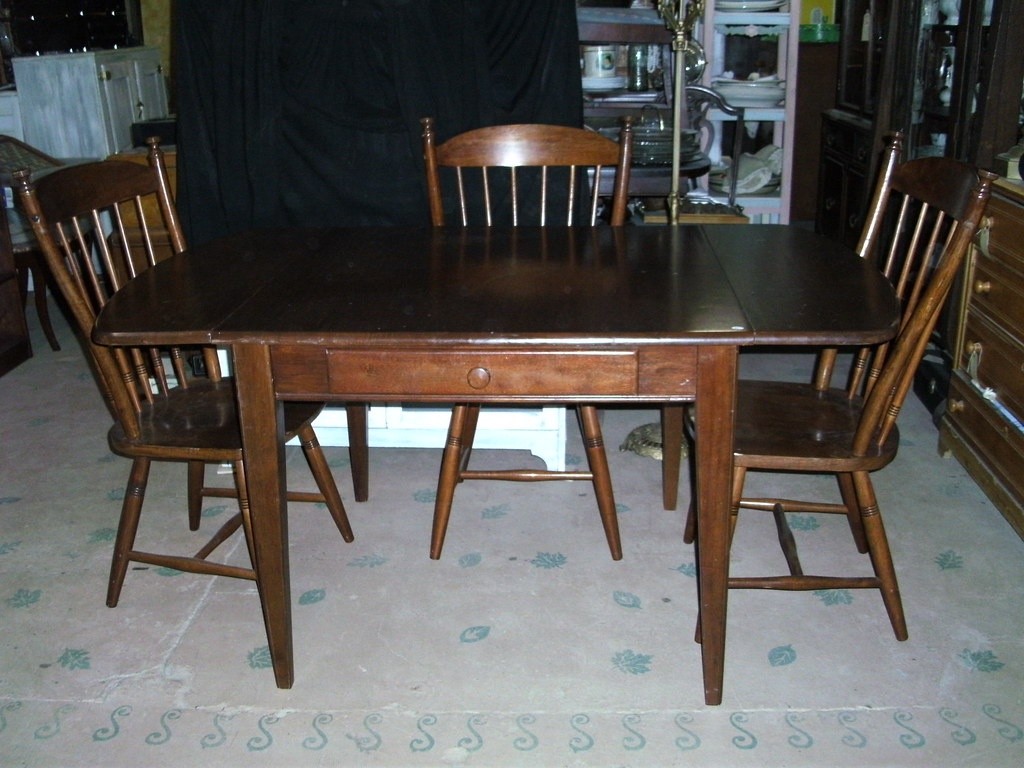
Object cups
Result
[580,44,616,76]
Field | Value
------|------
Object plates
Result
[597,125,705,169]
[714,76,786,87]
[580,79,621,93]
[713,1,786,12]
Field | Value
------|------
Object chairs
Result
[420,114,634,561]
[685,127,996,645]
[0,134,96,352]
[12,135,355,689]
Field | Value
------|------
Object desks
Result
[91,229,903,705]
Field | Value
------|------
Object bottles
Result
[628,42,647,89]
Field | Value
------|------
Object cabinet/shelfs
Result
[575,0,712,229]
[0,182,34,378]
[937,175,1024,542]
[209,349,571,475]
[11,46,170,281]
[814,0,1024,429]
[101,152,182,295]
[692,0,802,226]
